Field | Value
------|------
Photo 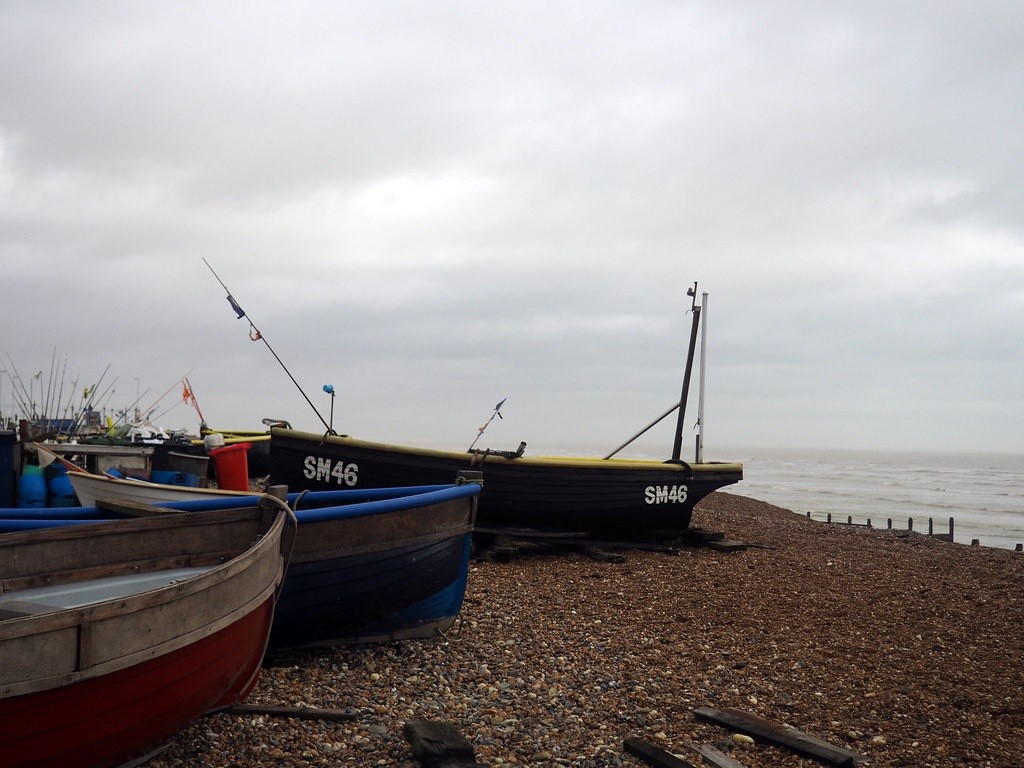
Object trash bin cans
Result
[212,442,252,490]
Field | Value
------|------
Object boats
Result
[199,420,271,438]
[268,283,743,543]
[65,468,279,509]
[1,485,291,767]
[193,433,270,476]
[0,482,482,660]
